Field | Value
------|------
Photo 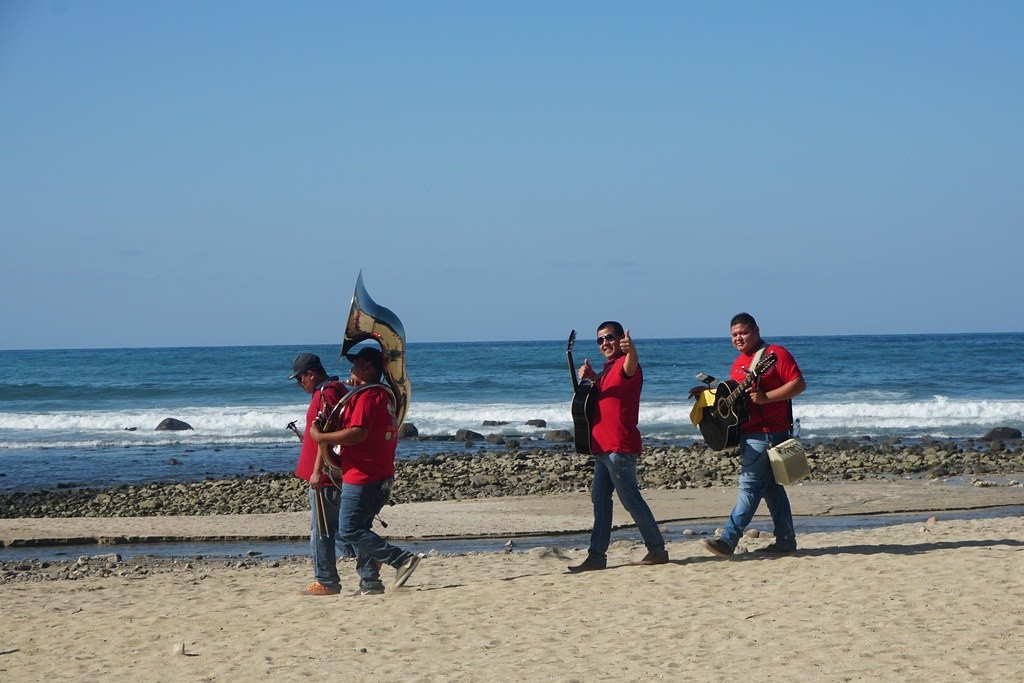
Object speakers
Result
[767,439,810,485]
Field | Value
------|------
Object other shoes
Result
[638,550,669,563]
[567,554,607,572]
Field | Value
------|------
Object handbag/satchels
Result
[768,438,809,485]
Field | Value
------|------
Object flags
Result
[338,266,411,438]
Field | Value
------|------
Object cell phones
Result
[696,372,718,384]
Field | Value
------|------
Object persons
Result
[568,321,670,573]
[688,313,806,558]
[288,348,424,595]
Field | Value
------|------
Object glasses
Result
[597,334,617,345]
[297,373,306,382]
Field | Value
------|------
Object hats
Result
[288,353,320,380]
[346,347,383,368]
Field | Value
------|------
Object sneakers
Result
[352,587,385,598]
[375,560,382,571]
[298,583,340,595]
[700,539,733,558]
[394,555,421,587]
[753,543,796,553]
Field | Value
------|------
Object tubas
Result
[314,267,412,479]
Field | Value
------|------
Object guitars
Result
[566,329,598,456]
[697,351,779,452]
